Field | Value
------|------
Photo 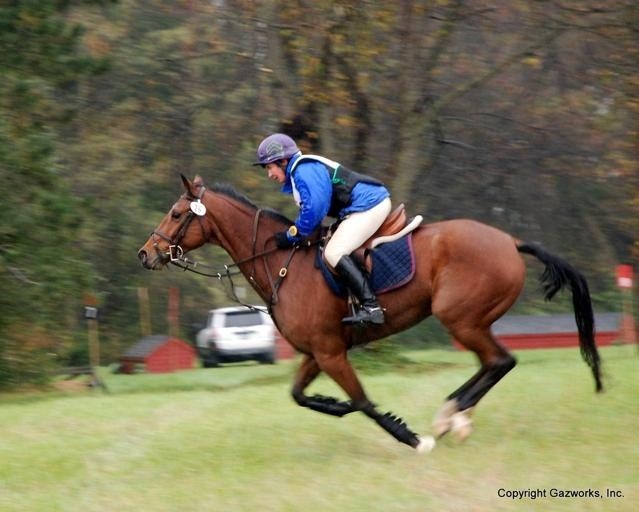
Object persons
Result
[250,133,393,327]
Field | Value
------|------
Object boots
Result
[334,254,385,327]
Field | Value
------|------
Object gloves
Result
[274,231,291,250]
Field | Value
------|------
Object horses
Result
[137,172,604,456]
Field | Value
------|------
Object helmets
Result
[251,132,299,167]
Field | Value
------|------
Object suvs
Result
[194,303,279,367]
